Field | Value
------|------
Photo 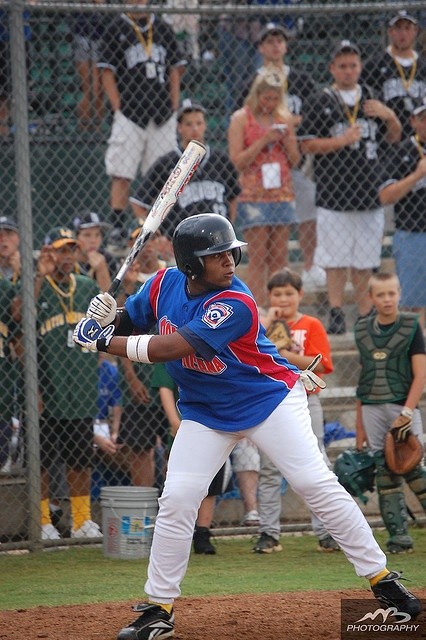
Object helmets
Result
[334,449,376,498]
[172,214,249,282]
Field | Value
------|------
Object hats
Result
[254,21,289,48]
[0,216,18,236]
[127,216,162,242]
[44,227,85,252]
[176,99,209,122]
[387,7,421,28]
[330,40,361,63]
[73,212,111,232]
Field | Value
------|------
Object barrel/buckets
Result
[99,485,161,562]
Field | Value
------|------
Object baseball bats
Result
[107,139,208,296]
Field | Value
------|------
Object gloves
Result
[389,407,413,445]
[302,354,327,393]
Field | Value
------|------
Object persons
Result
[152,360,231,556]
[234,439,260,527]
[254,268,343,554]
[164,3,201,67]
[366,7,426,160]
[122,219,183,487]
[65,12,105,127]
[96,346,120,501]
[380,98,426,337]
[128,98,240,238]
[234,20,317,267]
[355,272,426,555]
[13,226,103,539]
[297,39,403,334]
[97,1,186,223]
[73,213,421,634]
[76,209,113,290]
[0,217,23,472]
[228,64,305,303]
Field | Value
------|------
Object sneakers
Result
[1,417,21,477]
[354,307,377,325]
[301,265,354,293]
[240,510,261,527]
[370,571,422,622]
[385,537,415,556]
[252,533,283,555]
[117,603,177,640]
[326,307,347,335]
[193,526,217,556]
[413,96,426,116]
[316,536,343,554]
[69,521,104,547]
[39,525,69,549]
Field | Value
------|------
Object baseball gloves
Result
[72,317,115,353]
[86,292,117,329]
[387,406,415,444]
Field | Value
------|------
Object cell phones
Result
[273,124,288,130]
